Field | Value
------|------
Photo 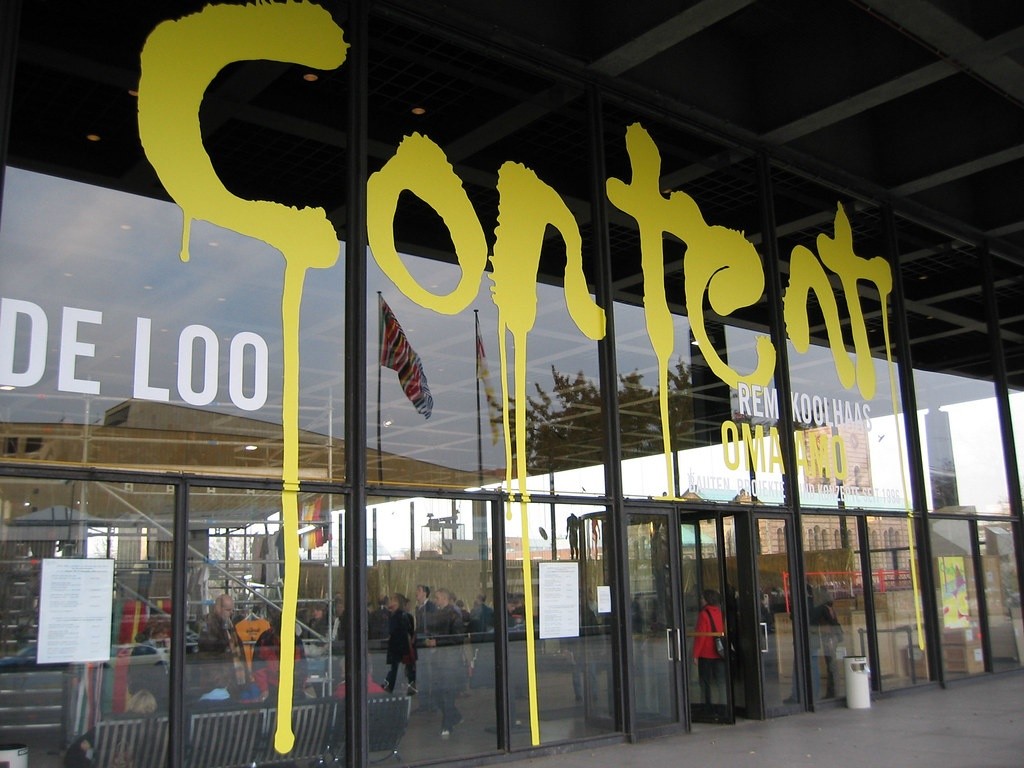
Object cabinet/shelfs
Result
[774,553,1024,684]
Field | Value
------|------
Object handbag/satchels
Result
[123,690,157,718]
[715,638,735,657]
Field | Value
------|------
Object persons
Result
[129,585,602,736]
[782,584,844,703]
[474,309,500,445]
[692,589,736,718]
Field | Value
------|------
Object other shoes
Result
[439,729,450,736]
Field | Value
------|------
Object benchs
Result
[85,691,415,768]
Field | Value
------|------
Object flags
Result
[377,291,434,419]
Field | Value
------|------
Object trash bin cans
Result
[842,654,872,709]
